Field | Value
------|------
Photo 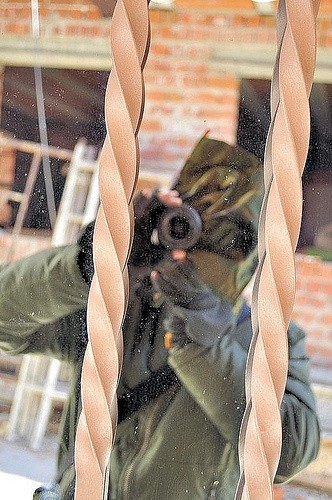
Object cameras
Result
[158,205,202,253]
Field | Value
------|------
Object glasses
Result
[193,209,259,260]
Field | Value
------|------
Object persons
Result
[0,139,321,500]
